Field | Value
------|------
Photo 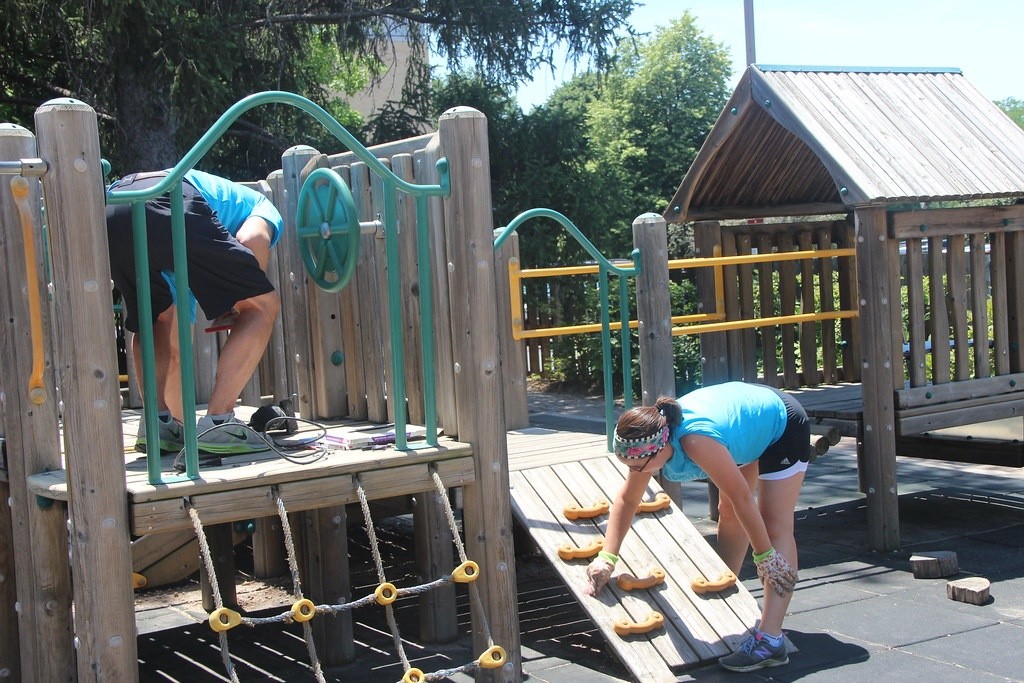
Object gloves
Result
[752,547,799,598]
[586,551,618,597]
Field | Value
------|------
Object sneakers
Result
[197,411,274,454]
[718,629,789,672]
[134,411,184,455]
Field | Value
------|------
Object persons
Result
[105,166,282,452]
[587,380,811,672]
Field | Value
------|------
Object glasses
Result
[627,455,654,472]
